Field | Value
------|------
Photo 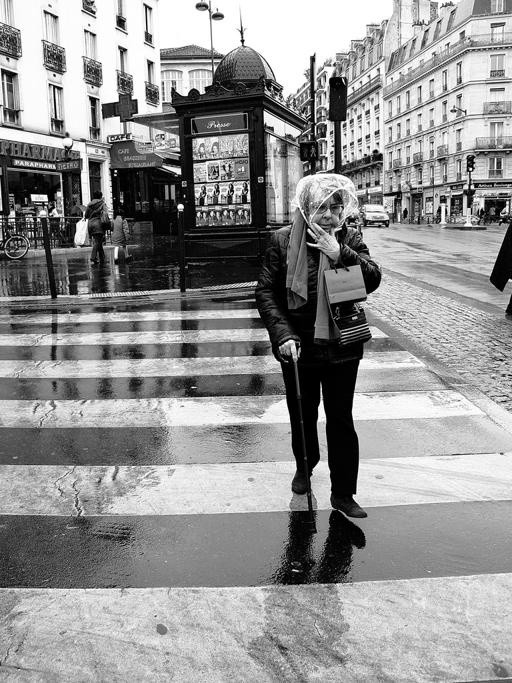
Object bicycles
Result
[0,223,31,262]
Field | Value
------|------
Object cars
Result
[358,203,390,228]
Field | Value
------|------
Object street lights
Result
[195,0,225,84]
[63,131,74,238]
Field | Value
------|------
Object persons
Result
[8,199,59,225]
[255,173,381,518]
[489,222,512,314]
[196,143,208,159]
[481,205,510,224]
[195,182,250,226]
[110,208,133,264]
[209,142,222,158]
[85,191,110,264]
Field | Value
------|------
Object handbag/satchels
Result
[100,211,111,231]
[333,308,372,347]
[324,264,367,305]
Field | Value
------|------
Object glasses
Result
[308,203,344,215]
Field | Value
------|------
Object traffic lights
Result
[299,140,320,162]
[467,154,475,173]
[326,74,348,123]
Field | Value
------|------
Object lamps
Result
[62,138,73,160]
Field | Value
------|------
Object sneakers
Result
[292,469,312,494]
[330,493,367,518]
